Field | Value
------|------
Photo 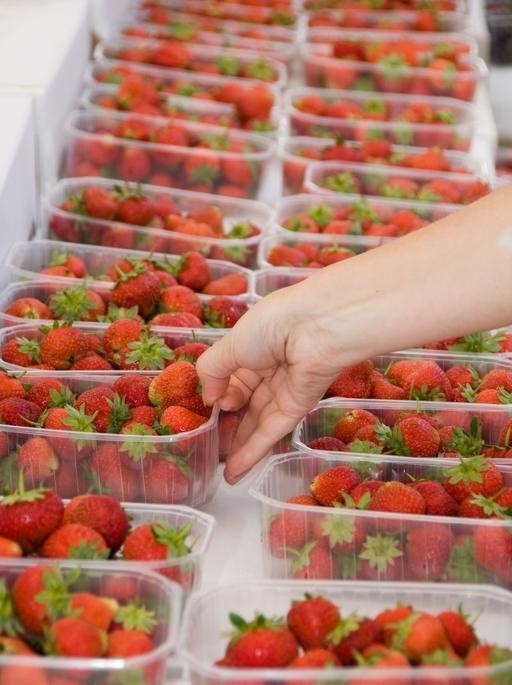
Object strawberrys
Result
[0,0,512,684]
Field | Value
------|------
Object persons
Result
[196,180,512,485]
